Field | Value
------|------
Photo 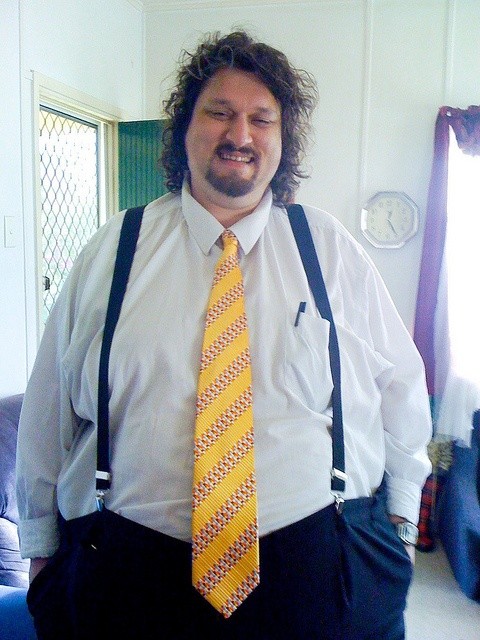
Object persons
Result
[13,17,435,635]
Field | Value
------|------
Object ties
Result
[191,230,261,619]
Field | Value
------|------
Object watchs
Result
[386,520,421,547]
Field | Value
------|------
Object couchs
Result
[438,408,480,604]
[1,393,36,640]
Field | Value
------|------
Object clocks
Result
[361,193,419,248]
